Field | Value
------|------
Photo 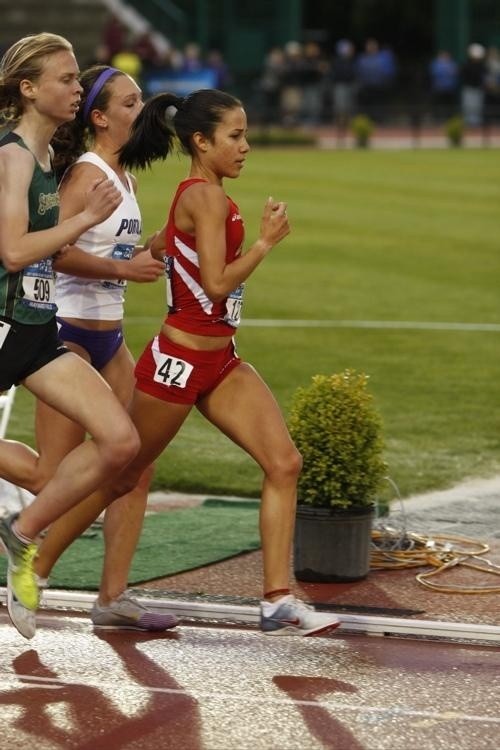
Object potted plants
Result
[283,369,393,585]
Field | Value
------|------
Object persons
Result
[86,28,499,147]
[1,32,347,644]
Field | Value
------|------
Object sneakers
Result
[0,511,38,609]
[93,593,179,632]
[8,562,48,639]
[260,594,342,636]
